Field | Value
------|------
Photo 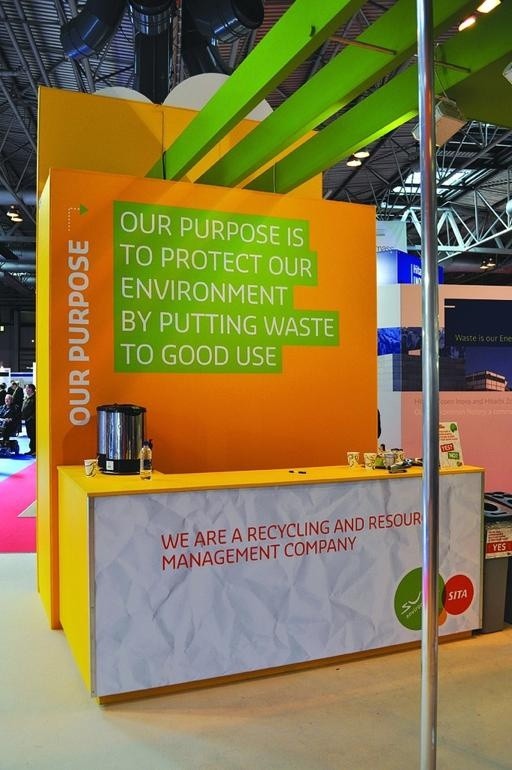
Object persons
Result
[0,379,39,457]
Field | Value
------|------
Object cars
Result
[84,459,98,478]
[363,452,377,471]
[381,450,405,470]
[346,451,361,468]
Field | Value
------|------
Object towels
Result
[484,492,512,634]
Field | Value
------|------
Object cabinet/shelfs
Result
[139,440,153,481]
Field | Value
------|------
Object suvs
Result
[102,406,147,475]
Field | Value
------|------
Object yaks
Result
[95,402,117,468]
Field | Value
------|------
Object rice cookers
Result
[388,461,407,473]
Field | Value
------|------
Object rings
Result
[289,470,307,474]
